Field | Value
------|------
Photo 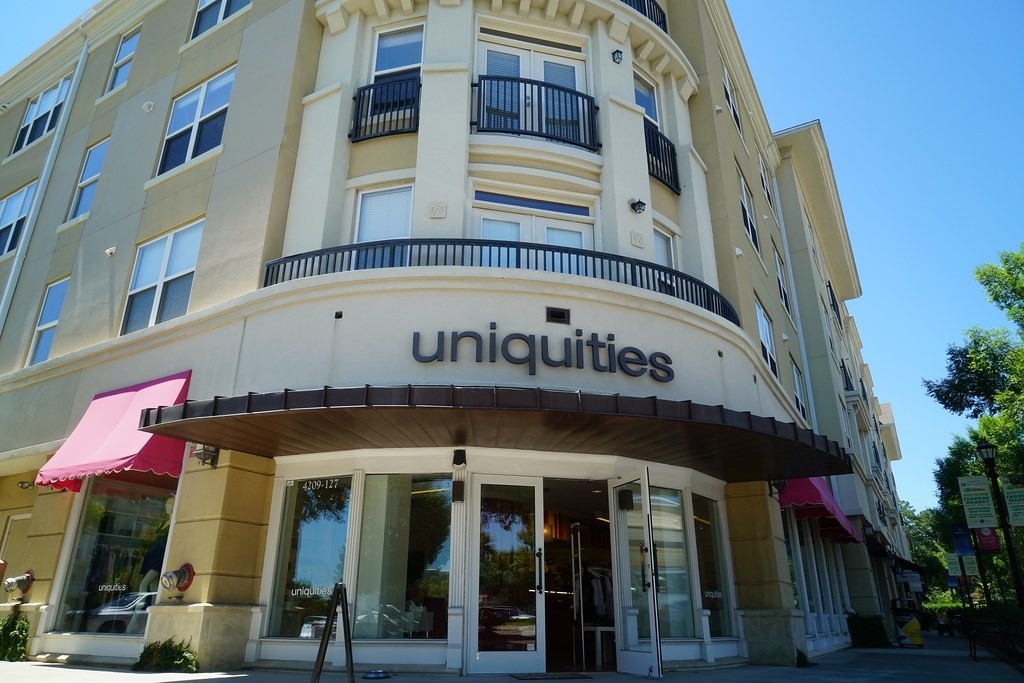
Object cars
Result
[629,567,689,634]
[952,615,961,629]
[87,592,156,634]
[300,614,337,640]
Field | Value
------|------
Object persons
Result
[125,498,175,634]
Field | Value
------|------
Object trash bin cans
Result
[890,597,924,649]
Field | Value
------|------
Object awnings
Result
[779,476,858,542]
[34,370,191,493]
